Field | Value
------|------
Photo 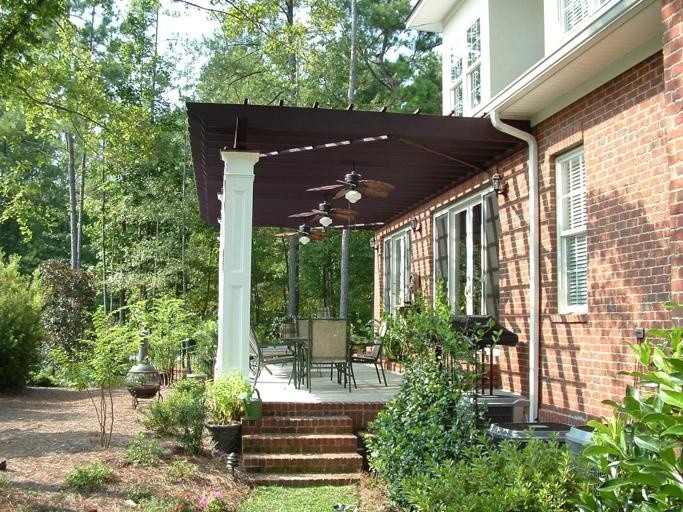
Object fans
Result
[273,161,396,241]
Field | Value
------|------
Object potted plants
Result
[203,371,252,454]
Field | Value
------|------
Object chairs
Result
[251,319,387,392]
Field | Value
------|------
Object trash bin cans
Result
[458,390,665,512]
